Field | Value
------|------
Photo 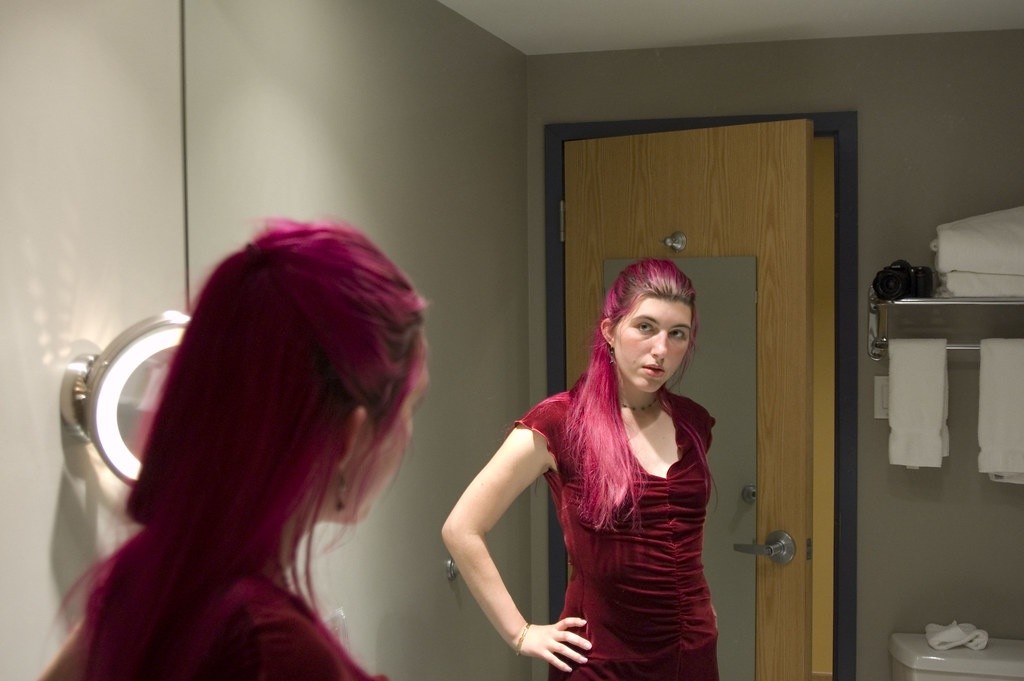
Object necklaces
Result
[618,398,657,410]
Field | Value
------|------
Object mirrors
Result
[179,0,1024,681]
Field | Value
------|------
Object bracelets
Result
[515,624,532,657]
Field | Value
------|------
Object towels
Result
[886,336,952,473]
[978,336,1024,485]
[931,203,1024,301]
[925,619,989,650]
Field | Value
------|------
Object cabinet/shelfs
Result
[867,285,1024,361]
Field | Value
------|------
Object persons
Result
[37,218,430,681]
[441,259,719,681]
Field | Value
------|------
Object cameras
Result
[872,258,932,302]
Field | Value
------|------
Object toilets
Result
[890,631,1024,681]
